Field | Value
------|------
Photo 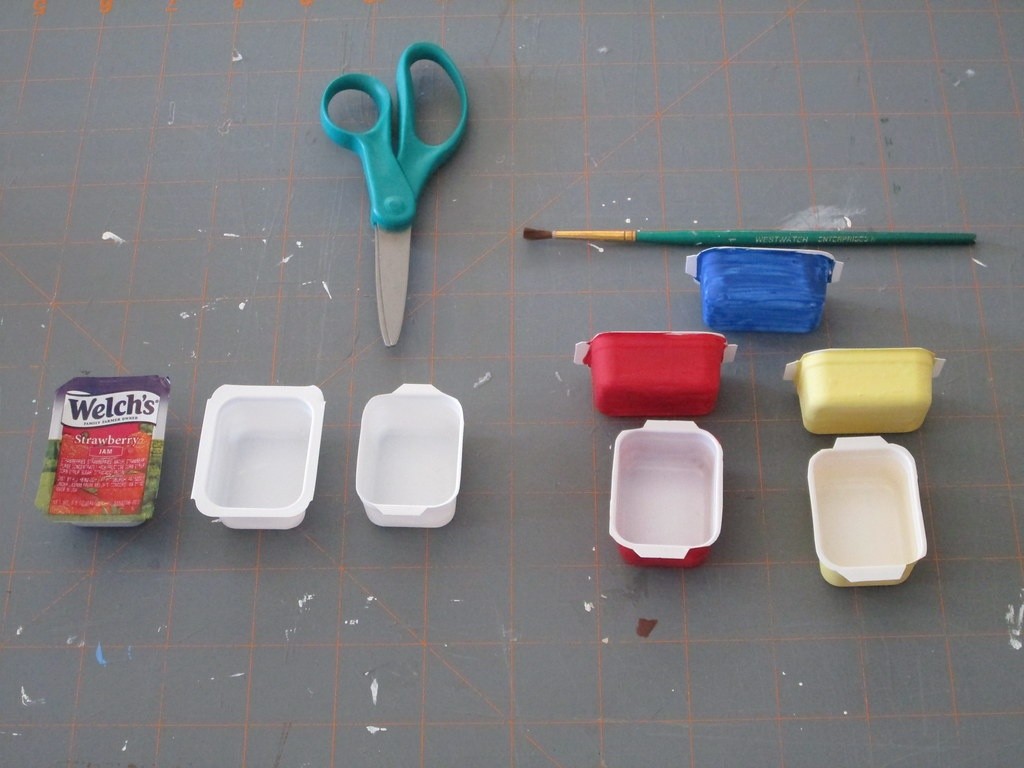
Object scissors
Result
[318,39,472,349]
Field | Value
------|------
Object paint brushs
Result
[517,221,981,248]
